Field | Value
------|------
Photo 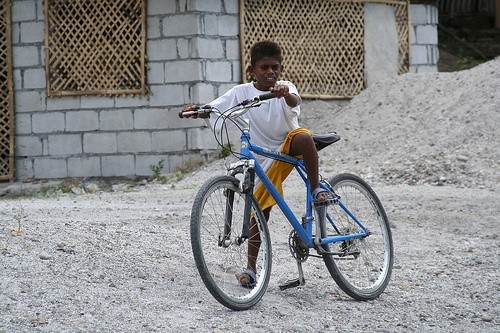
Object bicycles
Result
[177,88,394,312]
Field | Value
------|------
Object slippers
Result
[311,188,329,204]
[239,269,256,286]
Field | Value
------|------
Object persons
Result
[182,41,336,290]
